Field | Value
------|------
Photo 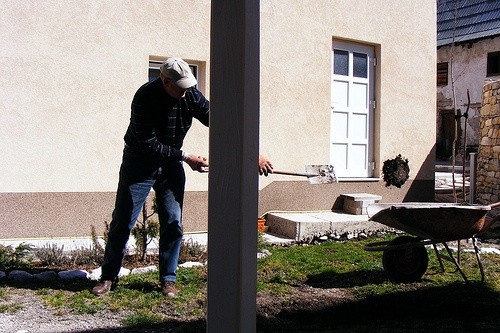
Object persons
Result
[93,57,273,297]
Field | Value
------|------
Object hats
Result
[159,58,198,90]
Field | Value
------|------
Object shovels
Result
[197,155,338,185]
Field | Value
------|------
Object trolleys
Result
[362,201,500,284]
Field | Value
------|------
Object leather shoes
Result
[91,279,113,296]
[161,282,177,299]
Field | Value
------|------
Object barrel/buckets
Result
[258,218,266,232]
